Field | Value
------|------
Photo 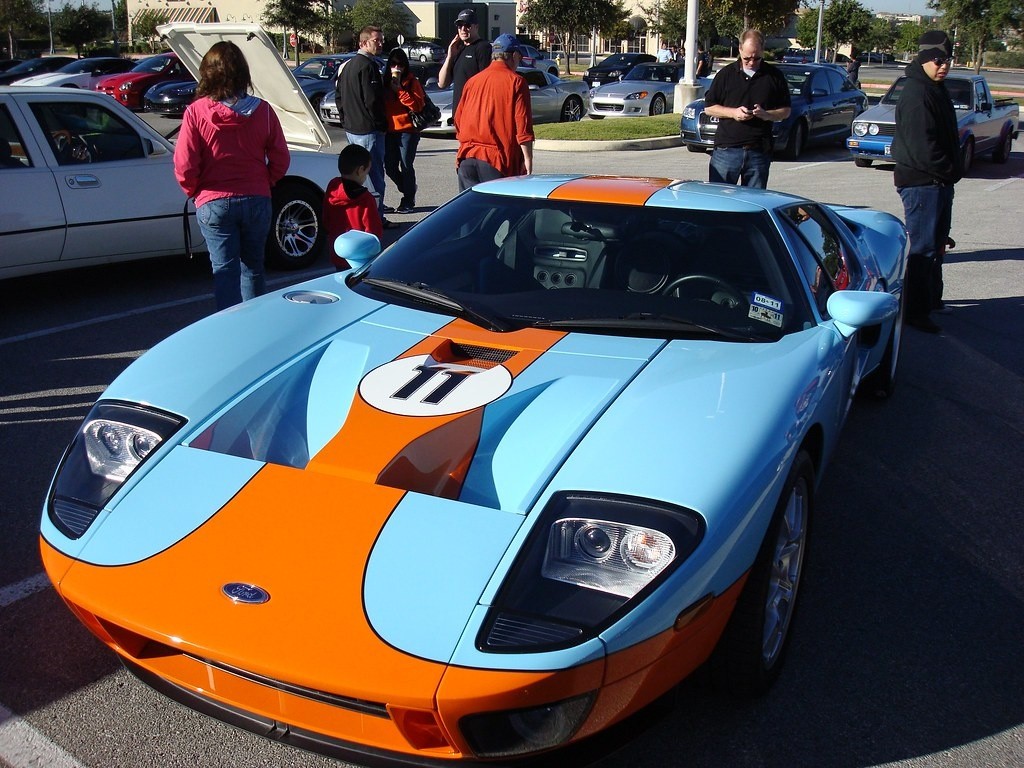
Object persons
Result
[846,54,861,83]
[334,25,400,228]
[454,34,535,236]
[384,48,425,214]
[704,29,791,189]
[321,145,384,273]
[656,42,713,77]
[173,41,290,311]
[439,9,493,116]
[890,30,963,333]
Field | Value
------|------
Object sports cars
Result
[40,174,912,767]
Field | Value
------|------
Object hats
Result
[918,31,953,64]
[454,9,477,24]
[490,34,529,56]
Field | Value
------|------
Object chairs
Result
[478,206,626,293]
[658,219,798,328]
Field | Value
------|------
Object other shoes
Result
[383,221,400,229]
[394,204,414,213]
[931,306,953,314]
[905,315,943,333]
[383,206,394,213]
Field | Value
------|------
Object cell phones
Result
[746,110,753,114]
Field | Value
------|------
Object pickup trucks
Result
[846,74,1019,172]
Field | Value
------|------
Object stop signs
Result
[549,32,555,43]
[290,34,299,47]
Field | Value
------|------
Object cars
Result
[587,63,684,120]
[783,50,828,64]
[856,52,887,62]
[582,53,657,90]
[519,45,560,78]
[320,61,443,124]
[291,54,386,118]
[0,22,379,279]
[836,54,853,63]
[419,67,591,133]
[389,41,445,63]
[680,63,868,159]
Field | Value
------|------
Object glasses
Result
[456,23,476,29]
[366,39,385,44]
[744,57,761,61]
[932,57,952,65]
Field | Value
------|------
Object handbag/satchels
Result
[400,71,441,132]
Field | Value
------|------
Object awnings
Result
[131,7,217,26]
